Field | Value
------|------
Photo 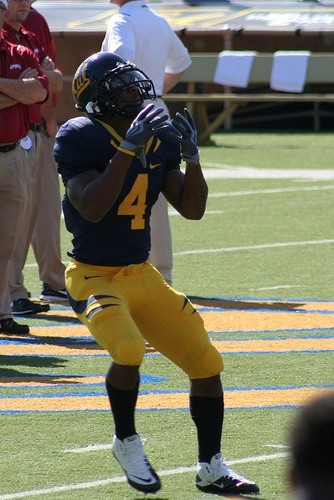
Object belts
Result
[157,96,161,98]
[0,143,16,153]
[29,120,46,132]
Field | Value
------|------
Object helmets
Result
[71,51,157,118]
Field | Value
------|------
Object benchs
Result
[162,53,333,147]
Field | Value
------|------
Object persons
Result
[100,0,192,289]
[287,386,334,499]
[54,52,260,495]
[0,0,68,334]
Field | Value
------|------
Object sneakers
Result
[195,452,259,493]
[0,318,29,334]
[11,297,50,315]
[39,283,69,301]
[111,431,161,493]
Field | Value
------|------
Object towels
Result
[269,49,310,94]
[213,50,257,88]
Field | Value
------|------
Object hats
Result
[0,0,8,9]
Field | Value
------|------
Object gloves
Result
[118,104,170,167]
[166,108,200,163]
